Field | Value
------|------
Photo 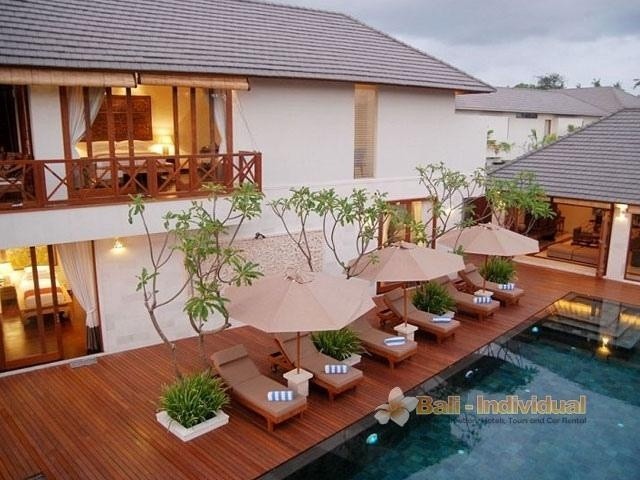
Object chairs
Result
[431,273,501,323]
[341,314,420,372]
[457,262,526,310]
[370,287,461,348]
[354,148,370,178]
[273,330,366,409]
[209,343,309,433]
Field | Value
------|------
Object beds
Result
[16,262,74,326]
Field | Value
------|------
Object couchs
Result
[75,137,175,186]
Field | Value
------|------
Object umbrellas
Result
[220,266,377,374]
[439,349,529,395]
[437,222,542,292]
[340,240,467,328]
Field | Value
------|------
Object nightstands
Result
[0,282,16,302]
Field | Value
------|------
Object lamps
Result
[151,135,174,156]
[110,238,130,254]
[599,332,609,353]
[0,263,15,285]
[615,202,628,224]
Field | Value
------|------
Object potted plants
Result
[412,284,458,323]
[153,365,233,445]
[307,329,372,369]
[479,251,518,290]
